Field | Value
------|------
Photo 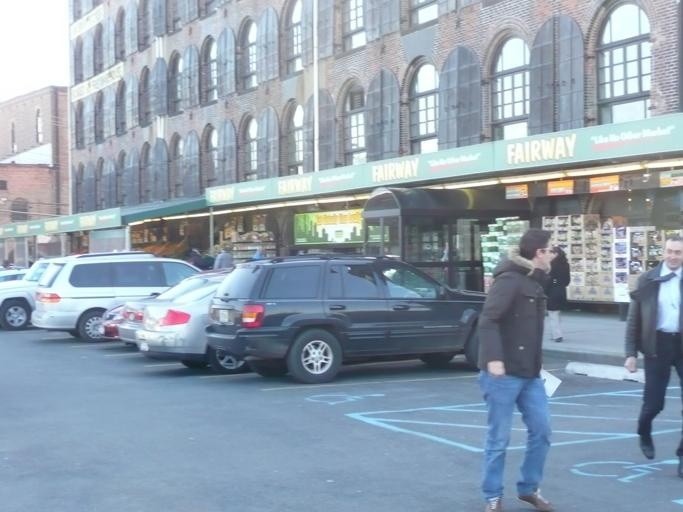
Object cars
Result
[98,266,234,368]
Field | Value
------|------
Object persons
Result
[1,260,12,271]
[625,234,683,478]
[254,245,268,260]
[546,247,570,342]
[477,230,557,512]
[213,241,235,269]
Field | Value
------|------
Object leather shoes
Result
[678,456,683,478]
[640,433,655,459]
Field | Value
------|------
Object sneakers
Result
[516,487,553,511]
[484,498,502,512]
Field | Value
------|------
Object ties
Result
[630,273,675,300]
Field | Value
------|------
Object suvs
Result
[205,254,487,384]
[0,258,202,342]
